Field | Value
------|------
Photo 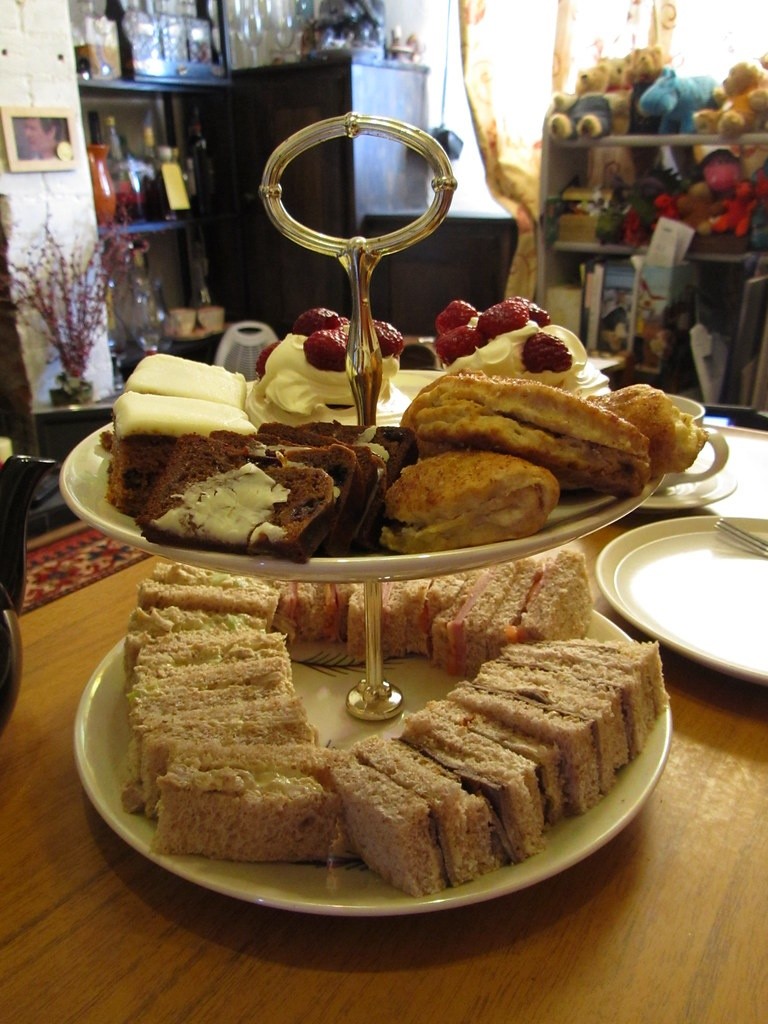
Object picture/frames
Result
[0,107,79,172]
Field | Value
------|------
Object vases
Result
[50,384,92,405]
[88,145,118,225]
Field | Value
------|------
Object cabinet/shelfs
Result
[535,118,768,413]
[198,59,517,340]
[68,1,243,233]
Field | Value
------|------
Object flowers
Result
[0,198,150,402]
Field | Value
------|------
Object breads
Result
[116,551,669,895]
[101,356,706,560]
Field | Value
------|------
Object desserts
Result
[432,297,613,402]
[244,308,406,425]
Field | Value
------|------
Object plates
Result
[595,515,768,687]
[72,610,673,918]
[639,458,738,511]
[59,369,666,586]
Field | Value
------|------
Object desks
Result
[0,425,767,1023]
[114,321,235,369]
[30,402,113,458]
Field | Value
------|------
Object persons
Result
[315,0,386,43]
[23,118,62,161]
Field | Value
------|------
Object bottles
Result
[86,105,222,225]
[70,0,223,83]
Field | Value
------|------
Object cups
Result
[654,392,729,496]
[171,307,225,335]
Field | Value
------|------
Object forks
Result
[714,518,768,558]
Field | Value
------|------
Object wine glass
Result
[224,0,315,69]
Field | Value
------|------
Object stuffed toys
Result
[596,149,768,248]
[548,47,768,141]
